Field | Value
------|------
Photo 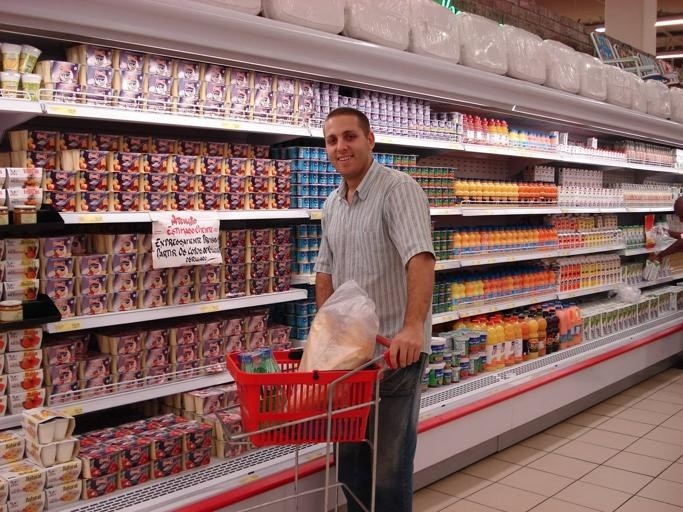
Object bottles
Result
[237,347,286,432]
[450,112,581,373]
[610,139,673,205]
[617,225,646,249]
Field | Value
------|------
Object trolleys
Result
[226,335,400,512]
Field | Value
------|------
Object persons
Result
[314,107,437,512]
[649,195,683,370]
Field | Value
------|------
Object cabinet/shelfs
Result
[0,24,682,512]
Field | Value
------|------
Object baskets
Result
[226,349,384,448]
